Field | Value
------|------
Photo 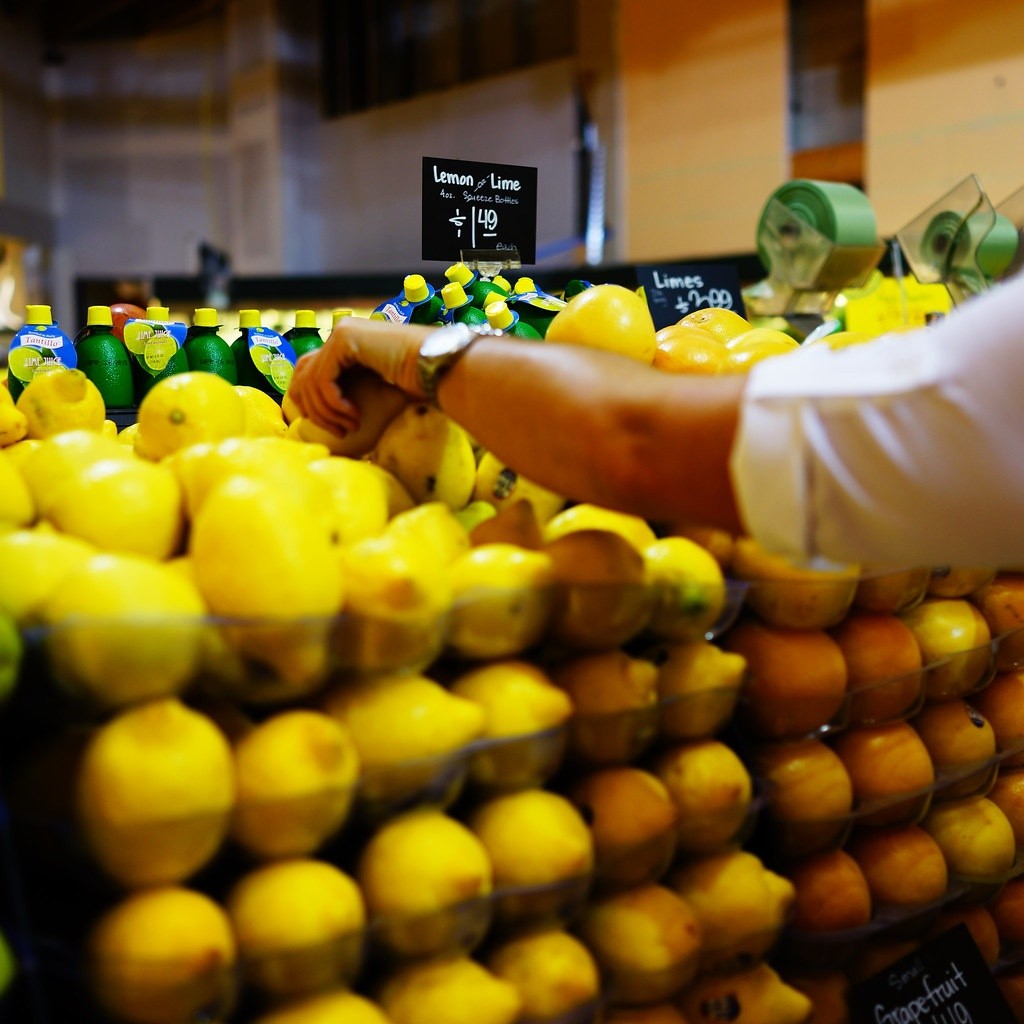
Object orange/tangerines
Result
[548,284,1024,1024]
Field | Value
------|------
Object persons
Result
[288,268,1023,571]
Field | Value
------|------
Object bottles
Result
[127,307,189,407]
[9,304,56,404]
[328,311,352,340]
[398,262,586,343]
[229,309,275,391]
[183,308,238,385]
[72,305,134,407]
[281,309,325,359]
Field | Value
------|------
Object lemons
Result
[0,278,817,1024]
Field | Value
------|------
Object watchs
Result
[417,319,484,413]
